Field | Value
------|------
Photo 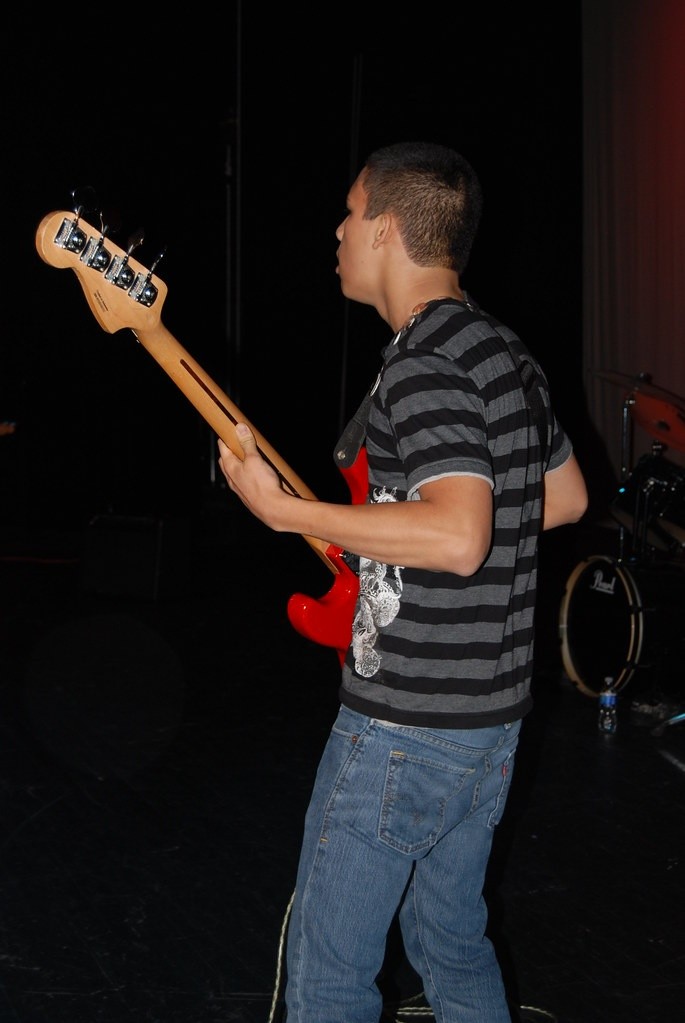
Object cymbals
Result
[584,367,685,410]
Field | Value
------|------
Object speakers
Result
[84,516,189,601]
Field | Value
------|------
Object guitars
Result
[35,208,370,665]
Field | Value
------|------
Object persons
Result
[217,144,589,1023]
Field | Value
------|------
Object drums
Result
[625,388,685,454]
[607,453,685,554]
[559,553,685,699]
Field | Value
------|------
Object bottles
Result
[599,676,618,735]
